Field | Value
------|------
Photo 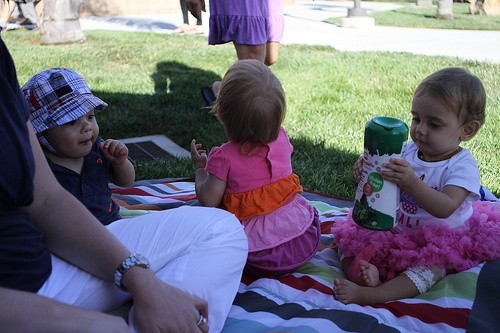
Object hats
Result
[21,68,108,135]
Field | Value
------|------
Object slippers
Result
[202,86,221,123]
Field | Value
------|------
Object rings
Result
[197,315,206,327]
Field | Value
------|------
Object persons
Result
[20,67,135,227]
[203,0,285,105]
[335,66,485,303]
[1,26,210,333]
[180,0,206,25]
[192,59,321,275]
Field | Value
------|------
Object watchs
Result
[114,252,149,292]
